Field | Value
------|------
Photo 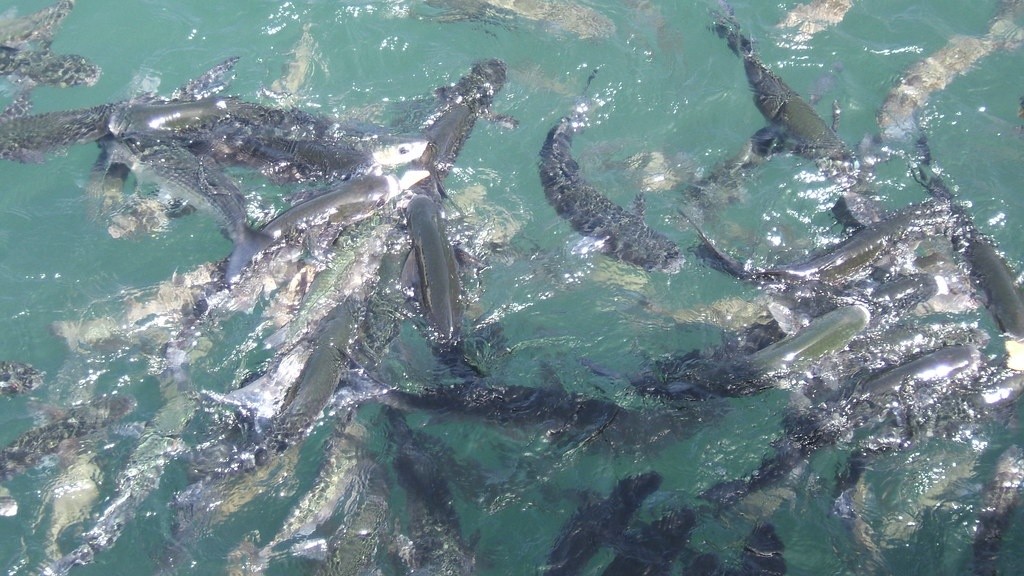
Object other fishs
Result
[0,0,1024,576]
